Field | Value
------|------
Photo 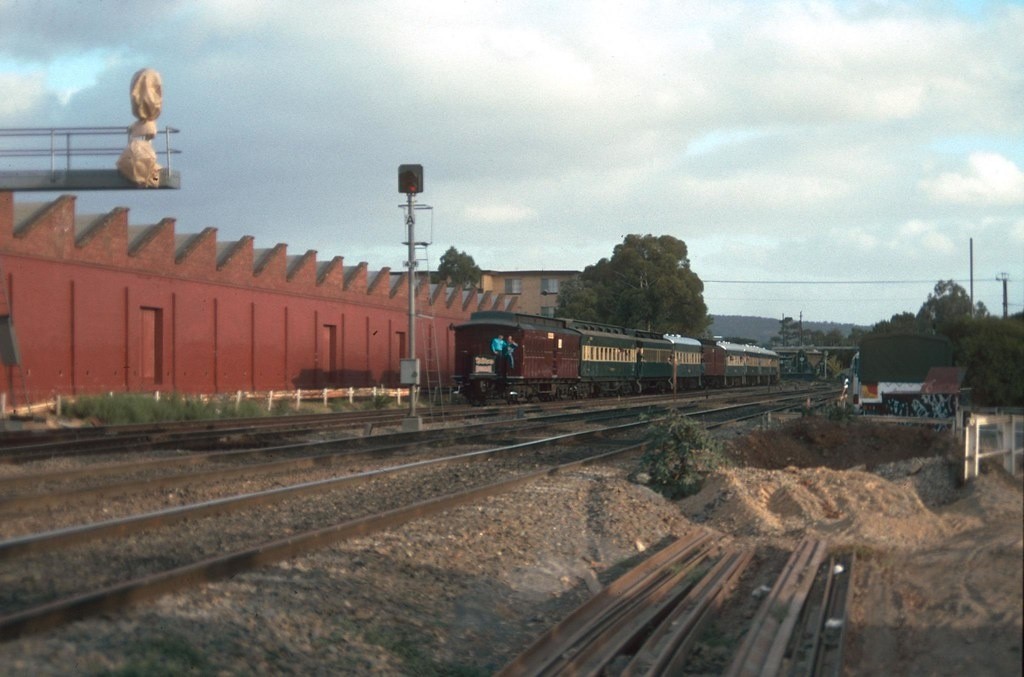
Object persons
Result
[490,334,518,376]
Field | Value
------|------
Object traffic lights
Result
[401,171,417,192]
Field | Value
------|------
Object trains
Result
[448,311,780,405]
